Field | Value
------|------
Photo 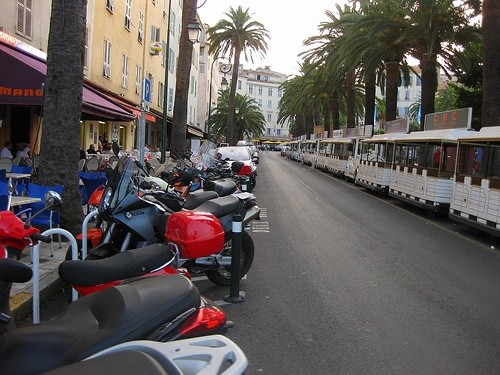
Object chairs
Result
[0,149,170,257]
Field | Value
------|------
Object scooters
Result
[1,139,261,375]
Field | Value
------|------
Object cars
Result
[236,140,260,164]
[215,145,258,190]
[258,140,291,157]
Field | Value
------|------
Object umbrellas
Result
[250,138,288,144]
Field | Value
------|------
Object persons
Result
[98,136,119,155]
[433,147,442,168]
[0,143,13,160]
[145,144,159,153]
[87,144,97,155]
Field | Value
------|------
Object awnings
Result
[152,109,205,138]
[113,98,156,123]
[0,42,135,122]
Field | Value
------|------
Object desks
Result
[10,196,42,263]
[6,172,32,197]
